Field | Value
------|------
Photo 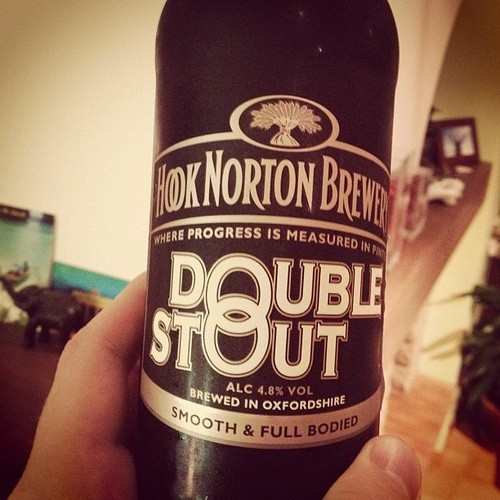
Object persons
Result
[6,269,425,500]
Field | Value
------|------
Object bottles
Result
[131,0,401,500]
